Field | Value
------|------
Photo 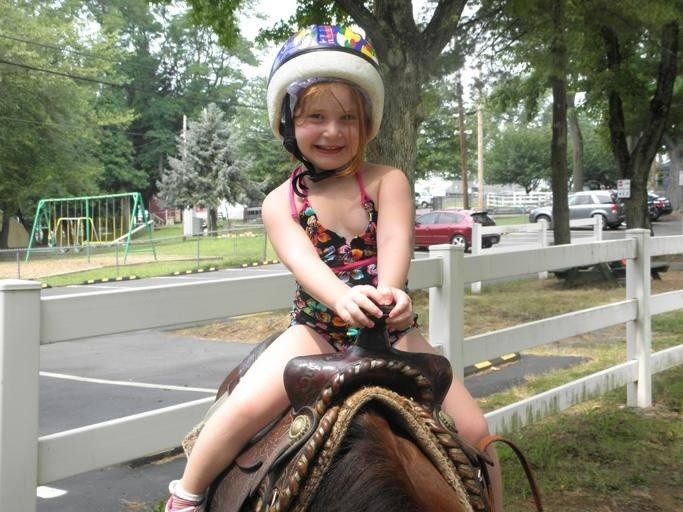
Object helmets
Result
[266,25,387,141]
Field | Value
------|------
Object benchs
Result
[612,262,669,277]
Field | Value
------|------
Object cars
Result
[412,208,500,253]
[530,188,625,231]
[613,193,673,224]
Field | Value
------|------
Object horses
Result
[212,324,472,512]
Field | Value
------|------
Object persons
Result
[162,24,507,509]
